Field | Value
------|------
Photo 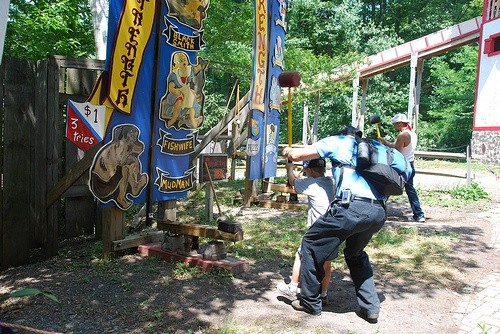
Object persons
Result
[278,158,335,306]
[281,126,387,321]
[376,113,426,222]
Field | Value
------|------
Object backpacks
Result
[350,130,414,196]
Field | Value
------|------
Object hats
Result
[335,126,363,136]
[391,113,409,123]
[299,157,326,175]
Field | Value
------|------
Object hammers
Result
[370,114,382,144]
[278,72,300,187]
[204,162,229,223]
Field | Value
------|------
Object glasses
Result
[302,171,308,176]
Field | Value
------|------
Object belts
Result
[354,196,386,205]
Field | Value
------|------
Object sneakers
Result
[277,281,300,301]
[319,294,329,307]
[413,214,426,222]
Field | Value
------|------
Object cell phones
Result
[342,190,350,204]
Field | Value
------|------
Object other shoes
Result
[291,299,322,316]
[354,306,380,323]
